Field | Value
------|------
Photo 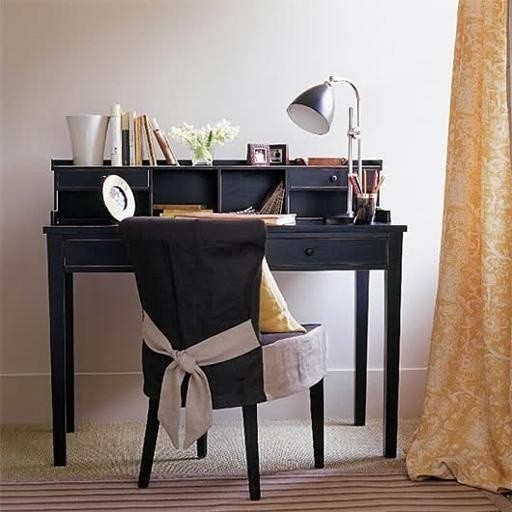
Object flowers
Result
[166,119,240,167]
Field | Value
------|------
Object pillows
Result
[258,256,308,333]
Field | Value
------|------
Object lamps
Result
[286,75,387,225]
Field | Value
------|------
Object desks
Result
[43,159,408,468]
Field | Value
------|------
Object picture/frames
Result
[248,143,270,164]
[269,144,289,165]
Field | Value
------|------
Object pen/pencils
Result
[347,168,386,193]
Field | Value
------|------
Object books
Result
[152,203,298,228]
[110,103,181,168]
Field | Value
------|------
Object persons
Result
[275,150,280,162]
[270,150,274,160]
[259,150,265,162]
[255,149,260,162]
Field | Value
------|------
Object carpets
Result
[0,418,412,482]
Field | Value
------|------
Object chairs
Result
[120,217,326,502]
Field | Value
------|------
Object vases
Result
[189,147,214,167]
